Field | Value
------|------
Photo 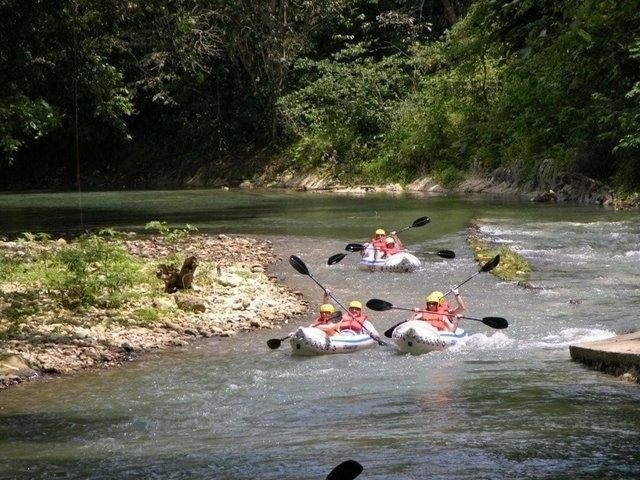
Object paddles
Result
[267,310,342,350]
[366,299,508,329]
[384,254,499,339]
[326,459,363,479]
[345,243,456,258]
[289,254,386,346]
[328,216,431,265]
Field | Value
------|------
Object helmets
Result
[385,237,395,246]
[320,304,335,315]
[375,229,385,240]
[348,301,362,314]
[426,292,445,310]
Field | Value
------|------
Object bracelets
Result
[455,293,459,297]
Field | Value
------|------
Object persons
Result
[433,285,465,321]
[360,229,402,260]
[324,300,380,340]
[411,293,462,333]
[309,288,335,328]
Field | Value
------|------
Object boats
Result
[289,319,468,355]
[359,243,421,272]
